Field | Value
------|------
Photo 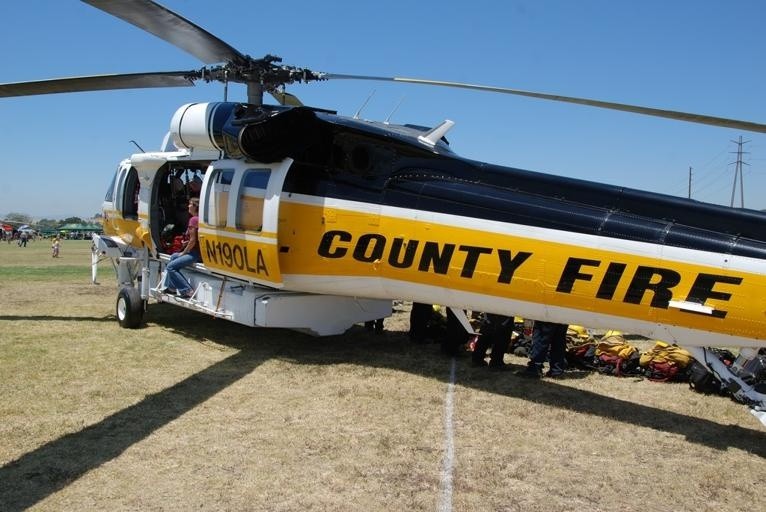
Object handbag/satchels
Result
[689,349,735,393]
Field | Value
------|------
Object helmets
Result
[566,325,590,338]
[600,329,623,340]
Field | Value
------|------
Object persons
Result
[52,234,60,257]
[163,196,199,298]
[364,318,384,335]
[513,320,568,377]
[20,231,27,247]
[472,313,514,371]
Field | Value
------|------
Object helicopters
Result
[1,1,765,348]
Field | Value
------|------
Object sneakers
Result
[471,359,543,377]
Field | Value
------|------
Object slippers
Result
[174,292,195,298]
[159,288,177,295]
